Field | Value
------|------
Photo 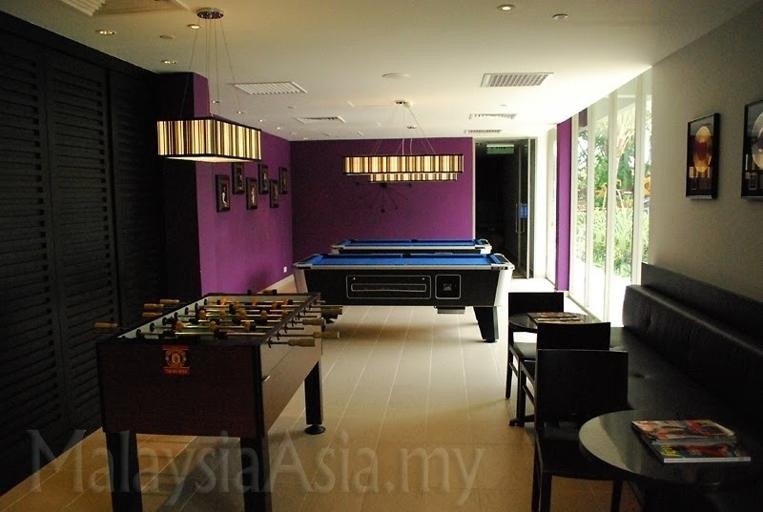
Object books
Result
[527,311,583,326]
[631,419,737,445]
[649,443,752,464]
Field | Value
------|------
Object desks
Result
[329,238,492,254]
[578,408,763,512]
[291,252,513,342]
[509,312,602,333]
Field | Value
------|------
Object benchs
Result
[610,286,763,512]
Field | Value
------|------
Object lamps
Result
[156,5,262,162]
[342,102,464,184]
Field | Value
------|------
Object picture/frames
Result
[216,175,230,212]
[279,167,288,193]
[246,177,257,209]
[270,179,279,208]
[686,113,720,199]
[741,100,763,198]
[232,162,245,194]
[258,164,269,194]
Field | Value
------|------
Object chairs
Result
[506,293,564,397]
[518,323,610,428]
[532,348,628,512]
